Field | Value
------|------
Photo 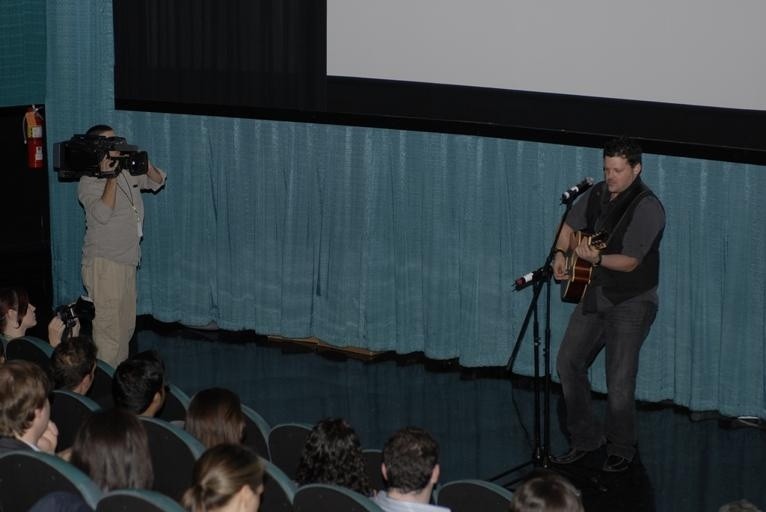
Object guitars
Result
[560,229,606,305]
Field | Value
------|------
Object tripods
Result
[488,285,542,486]
[500,271,567,491]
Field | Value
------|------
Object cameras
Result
[55,293,96,332]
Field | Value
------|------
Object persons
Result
[77,124,168,369]
[548,142,666,473]
[1,287,760,512]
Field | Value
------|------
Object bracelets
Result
[553,248,568,258]
[595,255,603,265]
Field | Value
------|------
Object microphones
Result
[562,174,596,202]
[517,265,556,288]
